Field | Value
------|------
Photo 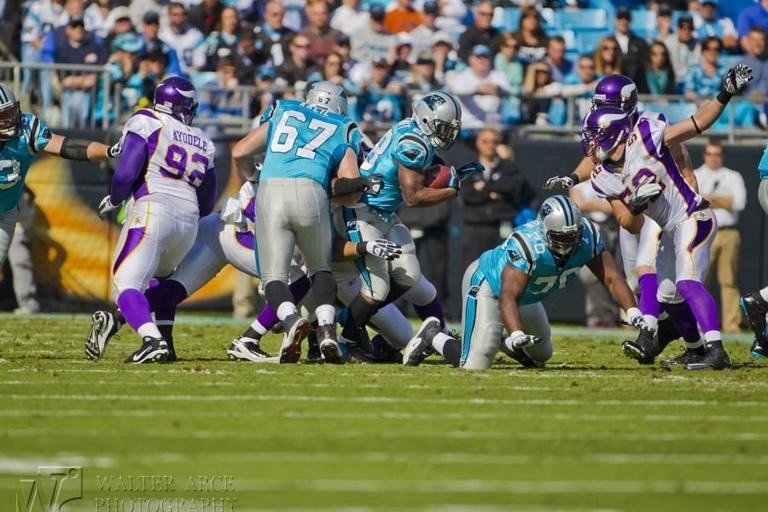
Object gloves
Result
[448,159,460,197]
[510,330,543,348]
[717,63,754,106]
[359,174,385,197]
[542,172,580,193]
[457,162,485,181]
[627,307,655,333]
[356,238,403,261]
[628,175,663,216]
[106,142,121,161]
[98,195,122,220]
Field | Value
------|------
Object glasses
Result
[171,13,185,16]
[706,48,720,51]
[704,152,722,156]
[268,12,284,17]
[603,46,617,51]
[617,15,630,19]
[292,44,309,49]
[480,140,496,144]
[504,44,520,50]
[652,52,666,56]
[476,12,494,16]
[70,23,84,28]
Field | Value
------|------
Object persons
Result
[85,172,402,362]
[333,91,461,359]
[20,0,768,129]
[0,83,122,270]
[402,194,654,369]
[739,147,768,356]
[456,127,534,262]
[582,63,754,371]
[85,77,217,365]
[232,80,362,364]
[399,199,452,318]
[331,207,413,356]
[541,73,699,364]
[693,142,746,335]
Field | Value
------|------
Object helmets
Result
[154,77,198,126]
[411,92,462,149]
[579,107,632,160]
[538,195,583,266]
[305,81,347,117]
[590,74,638,123]
[0,83,22,141]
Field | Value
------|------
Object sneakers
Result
[751,338,768,358]
[435,328,461,356]
[317,325,346,365]
[621,330,656,365]
[660,345,706,371]
[85,311,122,360]
[370,334,402,364]
[279,315,309,364]
[739,291,768,352]
[337,334,373,364]
[165,336,176,361]
[403,316,441,367]
[500,334,545,367]
[685,340,732,370]
[306,330,323,363]
[226,335,279,363]
[124,336,169,364]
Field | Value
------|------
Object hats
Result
[424,0,439,17]
[678,16,693,24]
[371,57,387,67]
[616,7,631,21]
[145,12,159,25]
[657,5,671,16]
[334,34,350,45]
[700,0,717,7]
[114,33,145,52]
[431,31,455,50]
[62,14,83,27]
[368,4,384,17]
[114,6,133,23]
[472,44,489,58]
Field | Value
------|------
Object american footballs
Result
[423,164,452,189]
